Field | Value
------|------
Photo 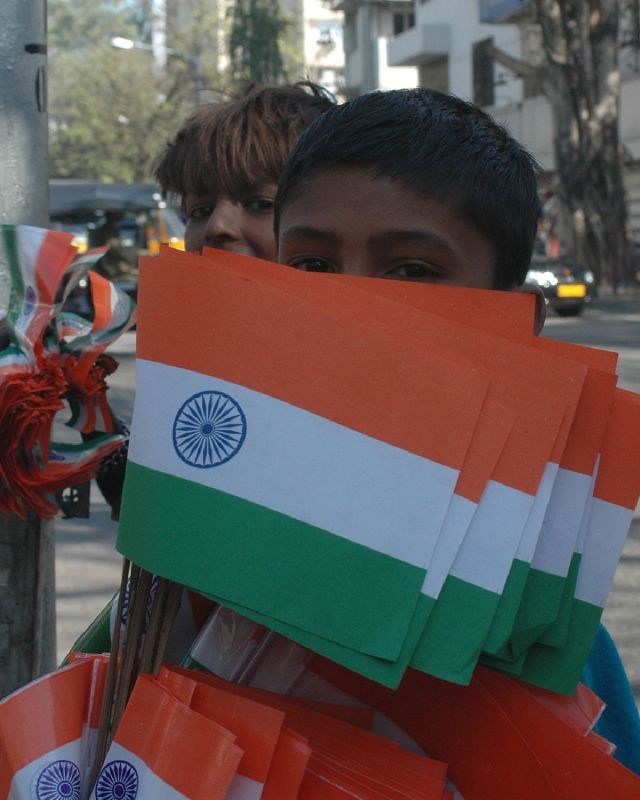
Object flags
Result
[0,223,640,800]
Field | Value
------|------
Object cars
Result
[47,179,187,308]
[522,253,598,320]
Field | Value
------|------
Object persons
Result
[157,77,345,268]
[271,87,640,775]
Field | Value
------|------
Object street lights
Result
[110,35,204,110]
